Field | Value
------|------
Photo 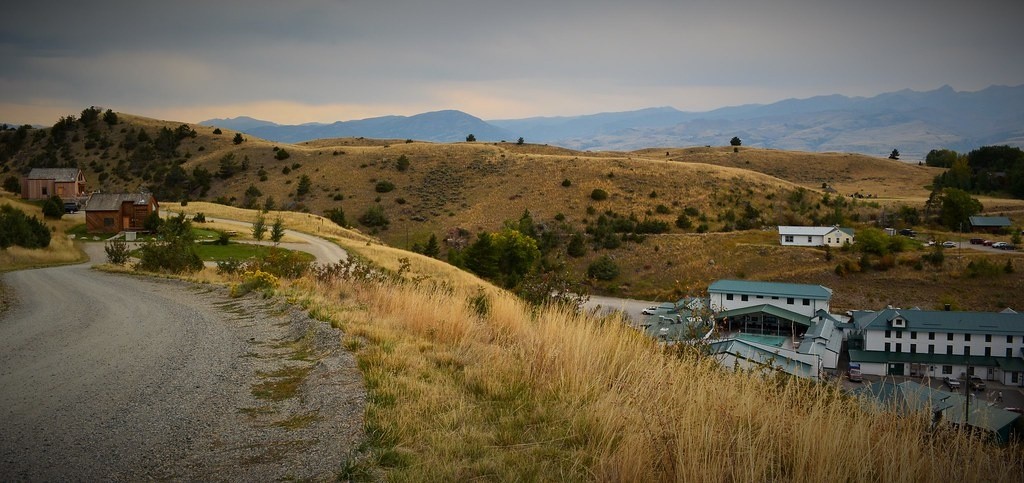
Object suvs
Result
[992,241,1008,248]
[62,202,81,214]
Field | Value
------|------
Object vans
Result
[970,238,986,245]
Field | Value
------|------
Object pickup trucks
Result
[848,368,863,382]
[968,375,987,391]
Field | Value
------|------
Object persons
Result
[997,390,1003,402]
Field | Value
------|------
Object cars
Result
[929,240,943,246]
[899,229,916,237]
[942,241,957,249]
[642,305,659,315]
[982,240,996,247]
[944,375,960,389]
